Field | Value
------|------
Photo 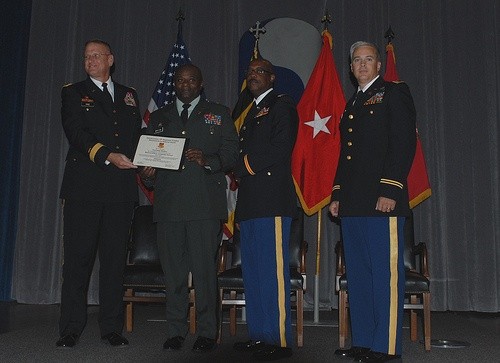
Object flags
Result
[137,40,206,203]
[292,30,347,216]
[220,49,258,247]
[385,45,431,209]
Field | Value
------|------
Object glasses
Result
[244,67,272,77]
[82,53,110,61]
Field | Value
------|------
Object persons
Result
[329,41,417,363]
[232,59,300,361]
[56,41,142,347]
[141,65,240,352]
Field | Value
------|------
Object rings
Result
[387,208,390,209]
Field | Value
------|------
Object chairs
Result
[124,205,195,335]
[335,209,431,351]
[217,207,307,347]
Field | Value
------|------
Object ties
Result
[102,83,114,106]
[248,100,256,113]
[351,90,363,109]
[181,104,192,126]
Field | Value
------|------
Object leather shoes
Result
[354,351,403,363]
[101,332,128,347]
[56,335,79,350]
[335,346,370,360]
[253,344,293,361]
[192,336,216,352]
[233,340,272,353]
[163,336,183,349]
